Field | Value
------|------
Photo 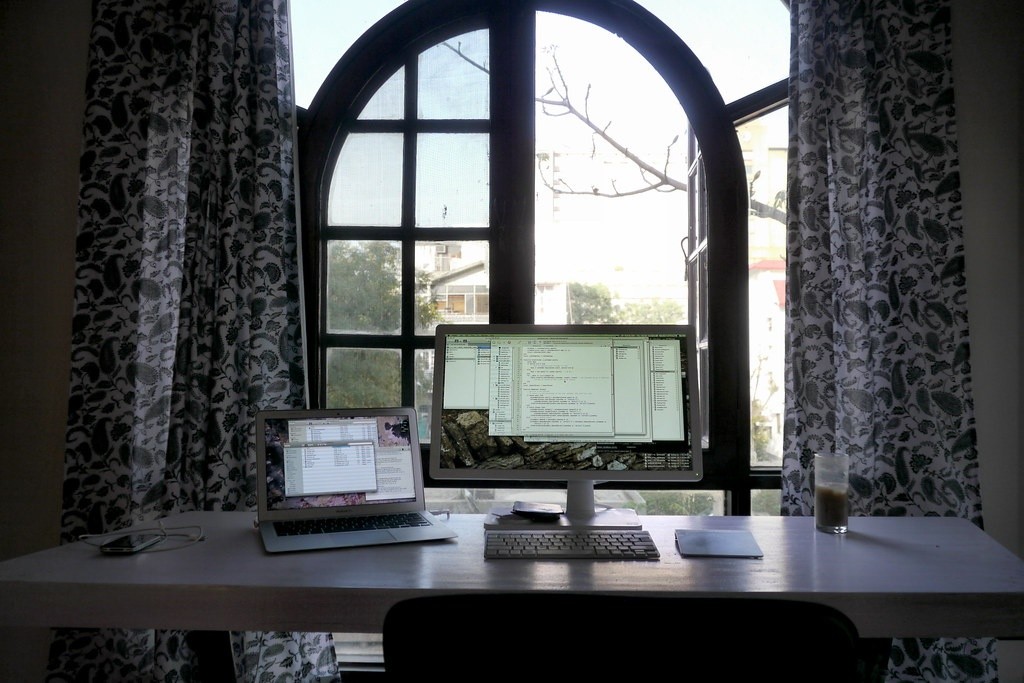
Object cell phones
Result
[99,534,161,552]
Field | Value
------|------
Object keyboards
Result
[482,531,660,559]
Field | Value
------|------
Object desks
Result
[0,510,1024,683]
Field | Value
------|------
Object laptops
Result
[255,408,464,550]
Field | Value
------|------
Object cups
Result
[814,452,850,534]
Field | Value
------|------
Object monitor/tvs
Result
[430,324,703,529]
